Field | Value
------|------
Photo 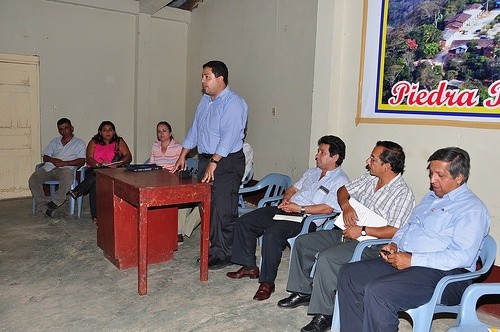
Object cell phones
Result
[380,249,391,256]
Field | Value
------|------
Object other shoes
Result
[197,255,233,270]
[178,233,185,246]
[44,201,58,219]
[70,189,82,200]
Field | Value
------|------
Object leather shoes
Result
[301,314,333,332]
[253,282,275,301]
[227,267,260,279]
[277,292,311,309]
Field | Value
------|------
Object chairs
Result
[31,163,80,216]
[446,282,500,332]
[331,235,497,332]
[77,167,88,219]
[260,210,337,273]
[237,172,291,217]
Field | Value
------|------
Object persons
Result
[169,60,248,269]
[29,118,86,218]
[178,127,253,244]
[150,121,183,170]
[70,121,132,224]
[337,147,491,332]
[277,141,415,332]
[227,135,350,301]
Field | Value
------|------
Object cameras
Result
[179,169,192,178]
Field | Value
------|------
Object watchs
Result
[361,226,366,236]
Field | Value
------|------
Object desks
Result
[93,169,212,295]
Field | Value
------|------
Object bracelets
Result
[301,206,305,215]
[209,158,218,164]
